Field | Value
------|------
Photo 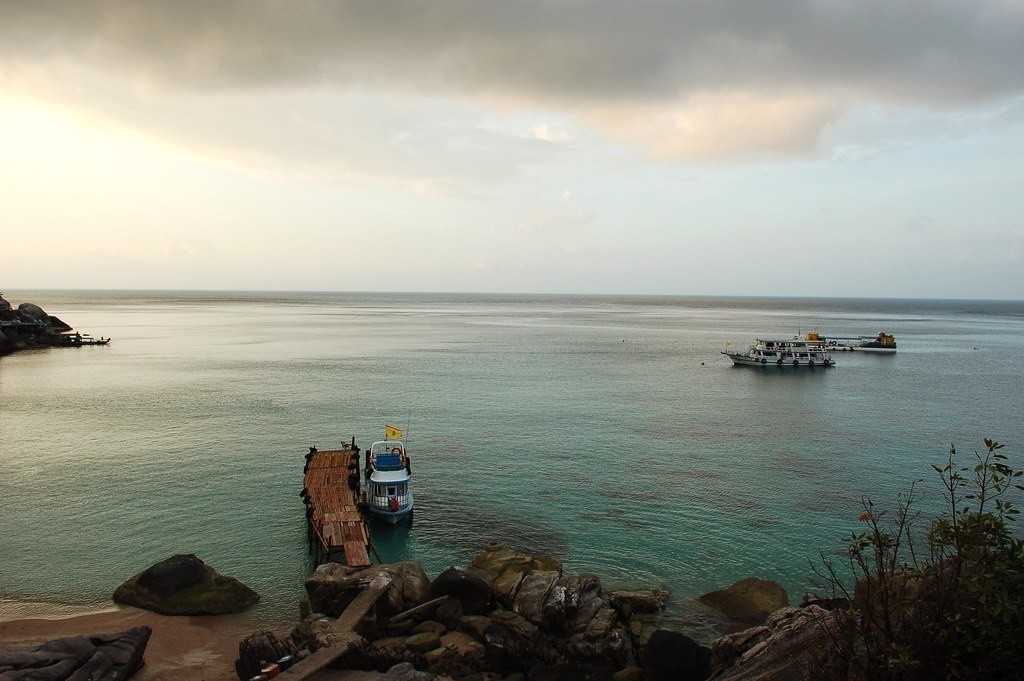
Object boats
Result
[364,424,417,525]
[719,332,836,366]
[826,331,898,353]
